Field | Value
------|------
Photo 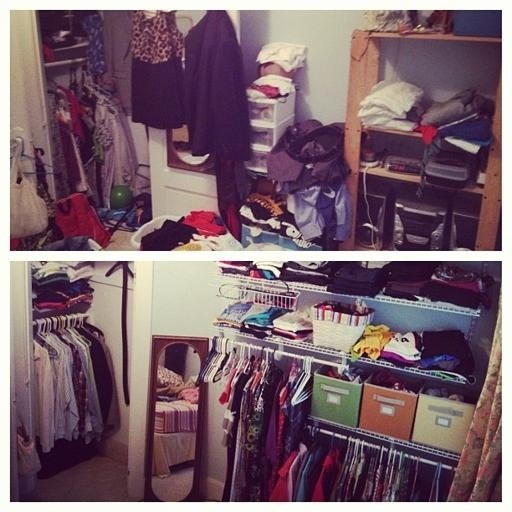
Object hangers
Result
[30,311,106,360]
[282,424,455,503]
[193,333,317,410]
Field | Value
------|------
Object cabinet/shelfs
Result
[336,28,501,255]
[10,7,241,209]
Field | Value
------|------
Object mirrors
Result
[144,334,210,500]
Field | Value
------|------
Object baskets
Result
[312,300,375,353]
[239,290,300,310]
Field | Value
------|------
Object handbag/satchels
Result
[10,177,48,238]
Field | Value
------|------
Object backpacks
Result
[56,192,111,247]
